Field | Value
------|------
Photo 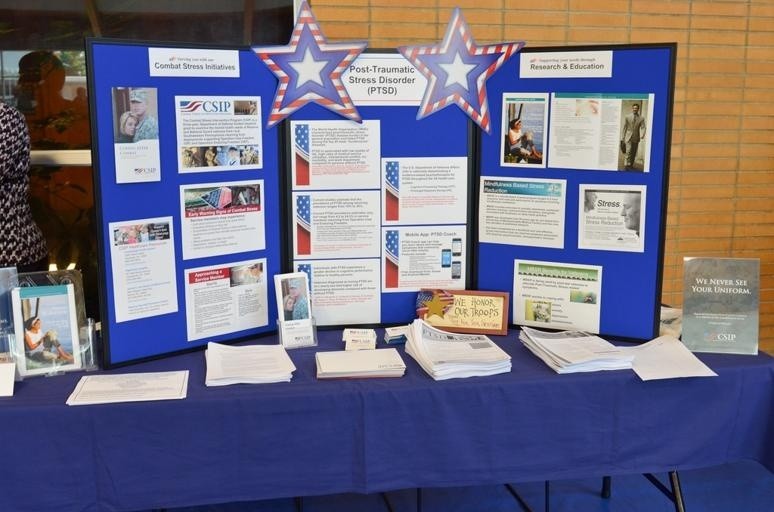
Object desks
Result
[0,300,773,508]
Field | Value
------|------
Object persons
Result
[583,191,597,213]
[113,110,140,144]
[126,89,158,143]
[283,295,296,321]
[619,193,640,237]
[116,224,155,243]
[238,264,263,286]
[288,279,308,320]
[507,118,542,164]
[182,146,258,167]
[621,103,646,170]
[0,101,49,272]
[13,50,100,324]
